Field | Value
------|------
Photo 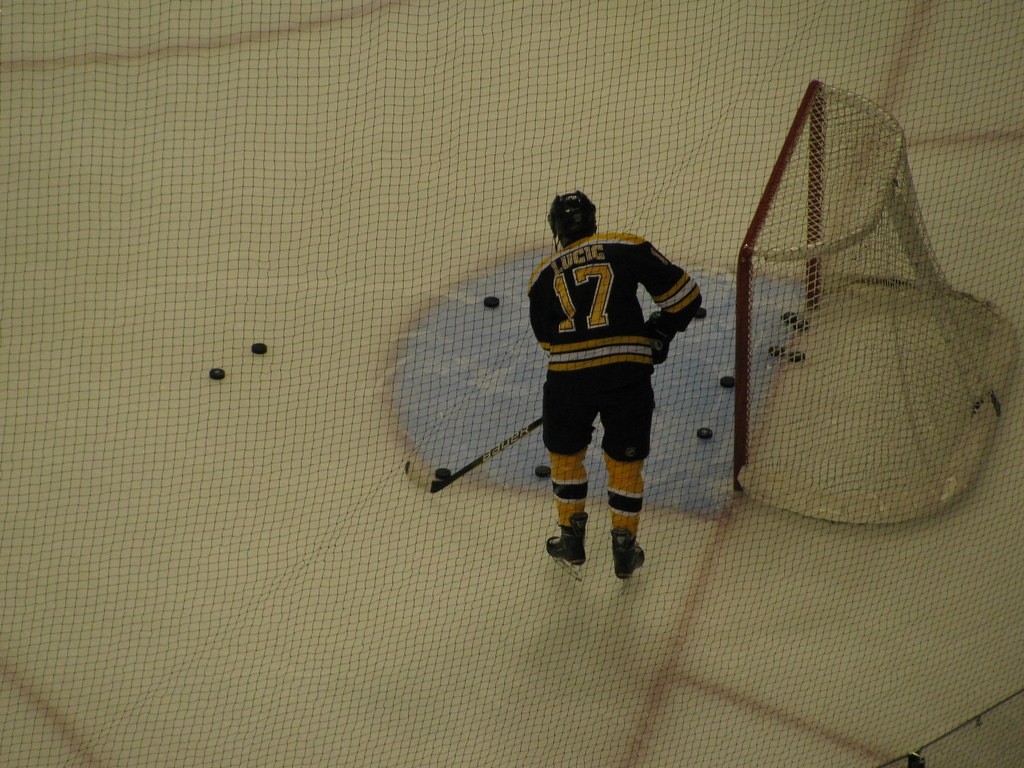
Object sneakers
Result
[546,511,589,582]
[610,528,645,595]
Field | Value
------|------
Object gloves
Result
[644,311,669,365]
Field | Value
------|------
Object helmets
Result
[547,190,597,247]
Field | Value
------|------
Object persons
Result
[526,190,703,595]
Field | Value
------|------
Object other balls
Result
[720,376,734,388]
[696,427,712,439]
[790,351,804,362]
[251,343,267,354]
[485,297,499,308]
[209,368,225,380]
[535,465,551,478]
[783,311,808,332]
[435,468,451,480]
[769,345,784,357]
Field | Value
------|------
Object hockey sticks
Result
[405,417,545,495]
